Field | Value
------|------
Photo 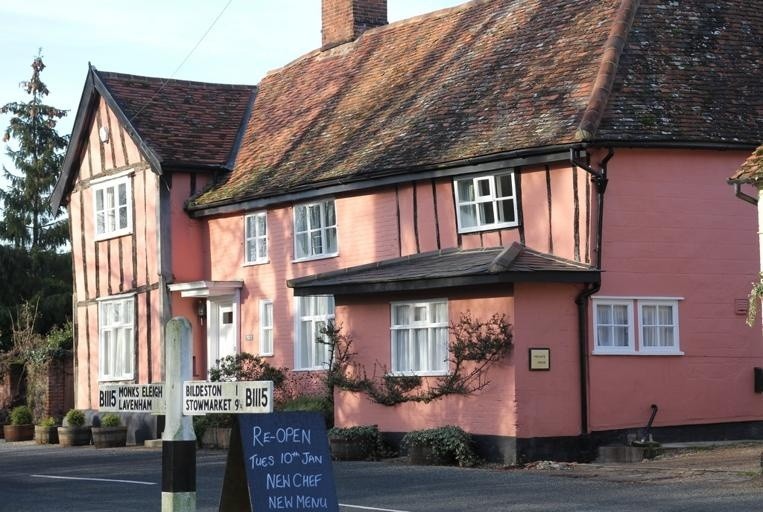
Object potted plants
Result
[2,405,127,448]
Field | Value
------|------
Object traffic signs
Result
[96,383,167,414]
[181,380,274,416]
